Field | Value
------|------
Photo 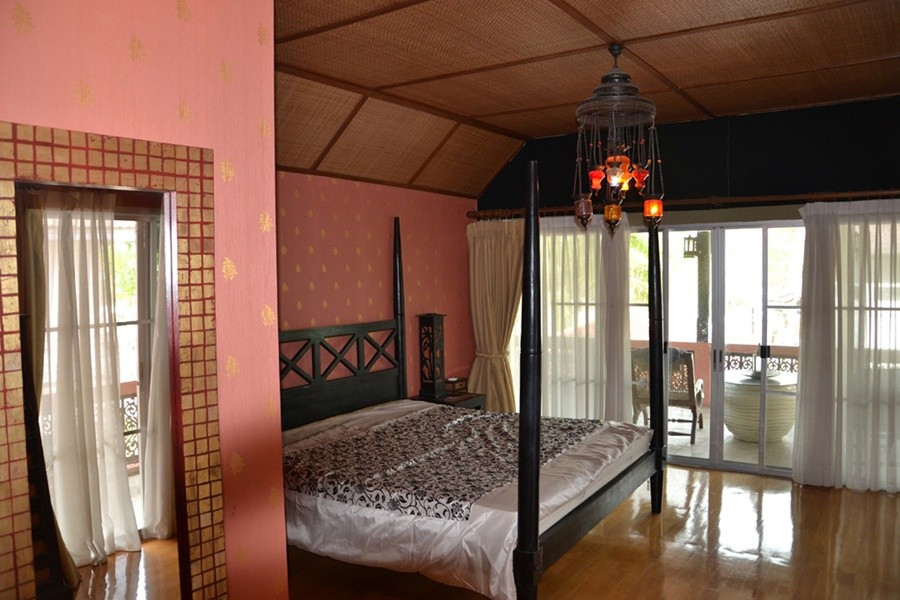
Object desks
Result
[721,370,798,445]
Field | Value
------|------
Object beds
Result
[273,157,670,600]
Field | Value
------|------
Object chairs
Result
[630,344,706,446]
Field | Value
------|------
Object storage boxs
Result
[447,376,471,396]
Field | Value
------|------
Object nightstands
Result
[445,392,489,411]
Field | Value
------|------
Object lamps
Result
[572,41,667,236]
[417,312,446,401]
